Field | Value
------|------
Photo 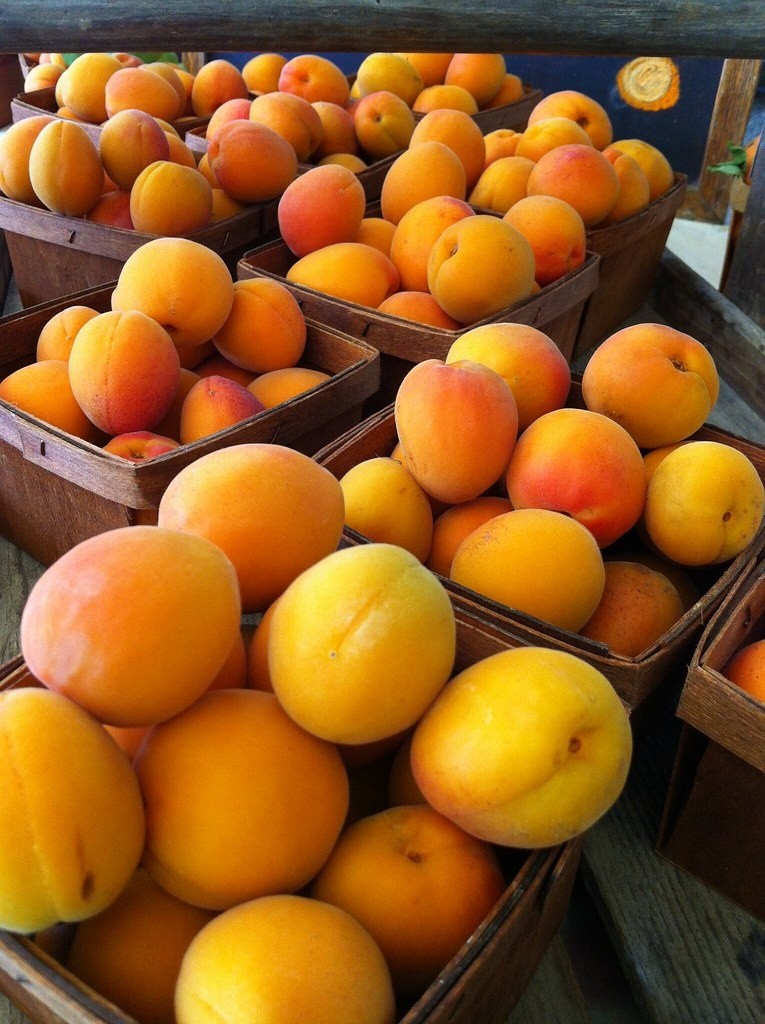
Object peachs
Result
[0,235,765,1024]
[0,49,760,331]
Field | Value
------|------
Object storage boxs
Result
[0,82,765,1024]
